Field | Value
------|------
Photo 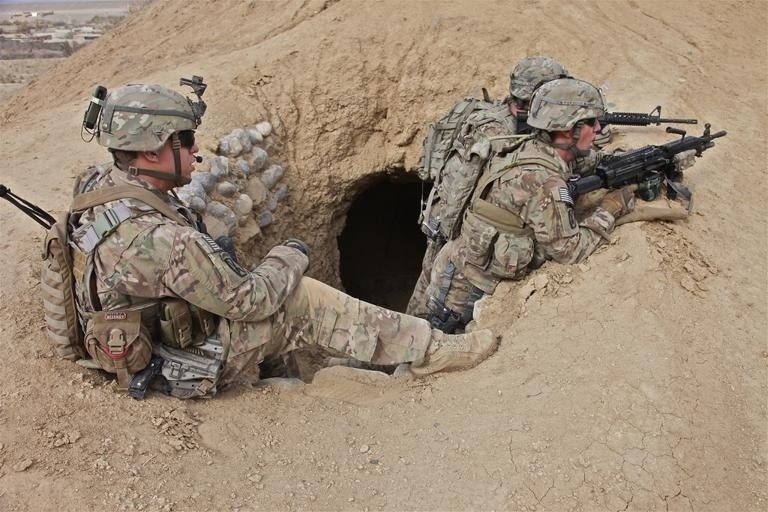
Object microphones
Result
[193,153,202,163]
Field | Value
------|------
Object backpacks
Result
[417,95,493,244]
[40,211,86,366]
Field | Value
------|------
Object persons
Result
[387,73,697,382]
[63,77,502,399]
[398,51,612,316]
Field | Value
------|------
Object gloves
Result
[600,186,637,219]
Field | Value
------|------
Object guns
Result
[566,125,728,201]
[129,358,219,399]
[516,106,697,134]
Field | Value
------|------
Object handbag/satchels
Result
[83,308,153,374]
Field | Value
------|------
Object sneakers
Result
[410,327,499,376]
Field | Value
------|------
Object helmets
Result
[510,56,607,131]
[81,76,209,152]
